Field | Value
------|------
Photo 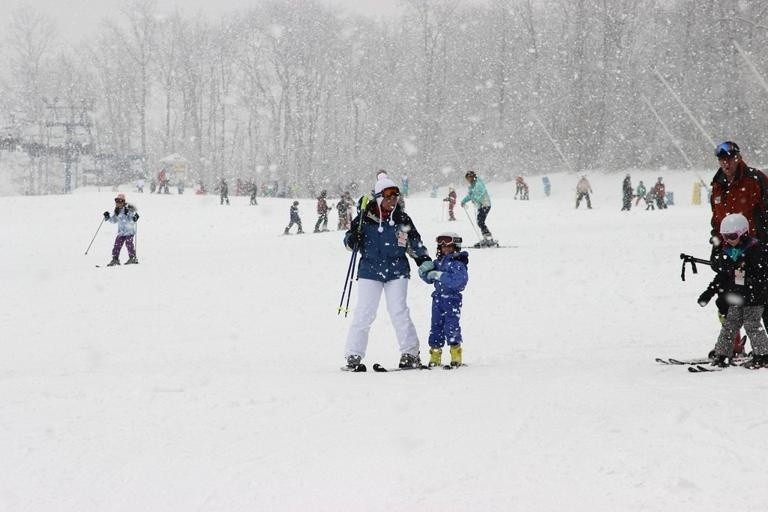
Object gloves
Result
[416,254,432,265]
[419,262,434,269]
[347,231,364,250]
[427,270,443,281]
[696,283,719,306]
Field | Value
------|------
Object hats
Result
[114,192,126,205]
[714,141,739,159]
[372,170,401,233]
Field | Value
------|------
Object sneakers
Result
[346,348,465,367]
[107,255,120,266]
[475,233,495,247]
[284,227,347,234]
[709,338,768,370]
[125,254,138,265]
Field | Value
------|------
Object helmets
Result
[436,231,463,253]
[717,212,750,238]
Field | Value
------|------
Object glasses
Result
[437,235,458,245]
[722,233,736,241]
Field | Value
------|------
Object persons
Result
[576,172,595,209]
[442,182,458,220]
[402,174,408,197]
[417,230,468,368]
[515,173,525,199]
[708,141,767,358]
[312,189,330,233]
[336,197,348,231]
[698,213,768,369]
[543,174,552,196]
[621,174,670,211]
[282,202,304,234]
[104,192,143,266]
[460,169,496,246]
[147,166,285,204]
[523,185,530,201]
[343,170,435,369]
[342,191,357,225]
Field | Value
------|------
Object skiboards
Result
[656,352,768,372]
[341,363,469,371]
[460,245,518,249]
[95,262,140,268]
